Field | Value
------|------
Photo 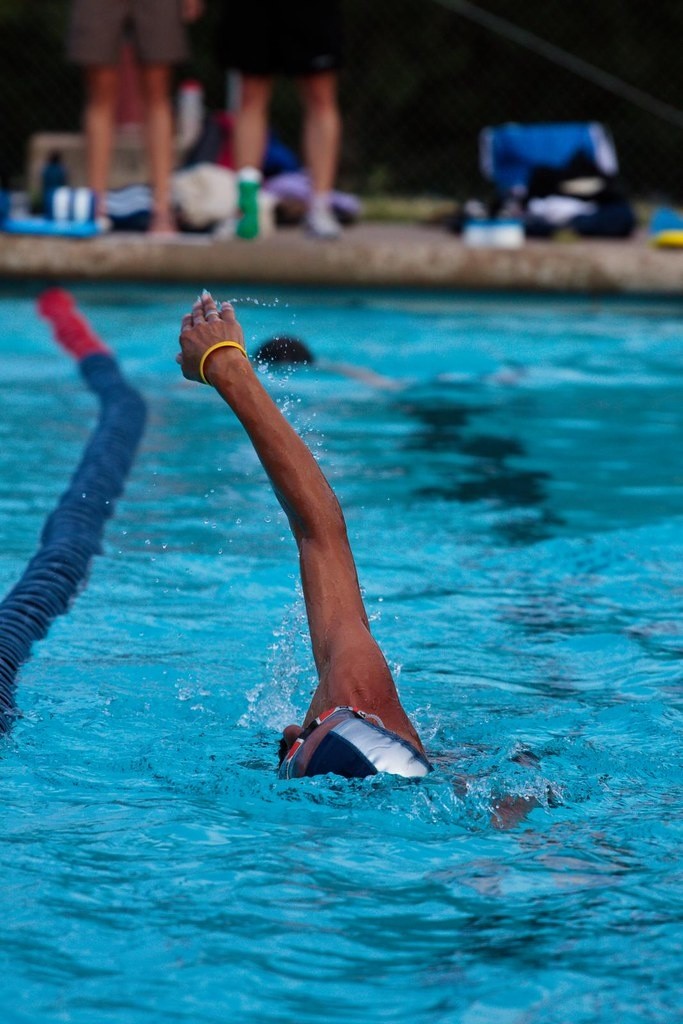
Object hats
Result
[304,717,435,781]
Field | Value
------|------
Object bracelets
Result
[200,341,248,386]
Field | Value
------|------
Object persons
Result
[255,334,314,367]
[200,0,346,241]
[64,0,198,240]
[175,289,434,780]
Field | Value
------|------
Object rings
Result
[205,310,219,319]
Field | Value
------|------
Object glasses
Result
[279,703,384,782]
